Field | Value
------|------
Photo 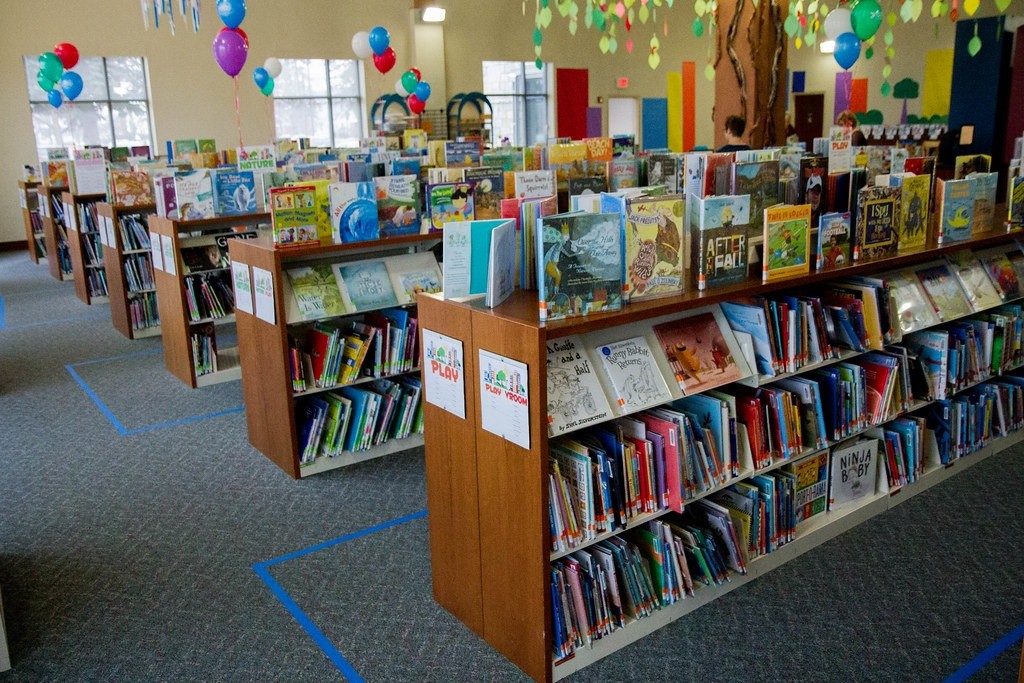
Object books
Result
[105,146,160,329]
[153,140,276,378]
[30,144,108,297]
[269,142,780,465]
[445,168,1024,666]
[778,116,943,176]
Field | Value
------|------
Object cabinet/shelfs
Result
[60,183,110,305]
[228,235,443,481]
[418,172,1024,683]
[37,182,74,282]
[95,201,163,342]
[147,211,271,390]
[17,178,47,264]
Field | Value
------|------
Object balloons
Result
[825,0,884,70]
[37,43,83,108]
[254,58,281,96]
[395,67,431,114]
[351,26,395,75]
[212,0,249,77]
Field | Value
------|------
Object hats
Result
[806,173,823,193]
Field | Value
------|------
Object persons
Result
[825,235,845,266]
[715,115,753,153]
[804,173,822,228]
